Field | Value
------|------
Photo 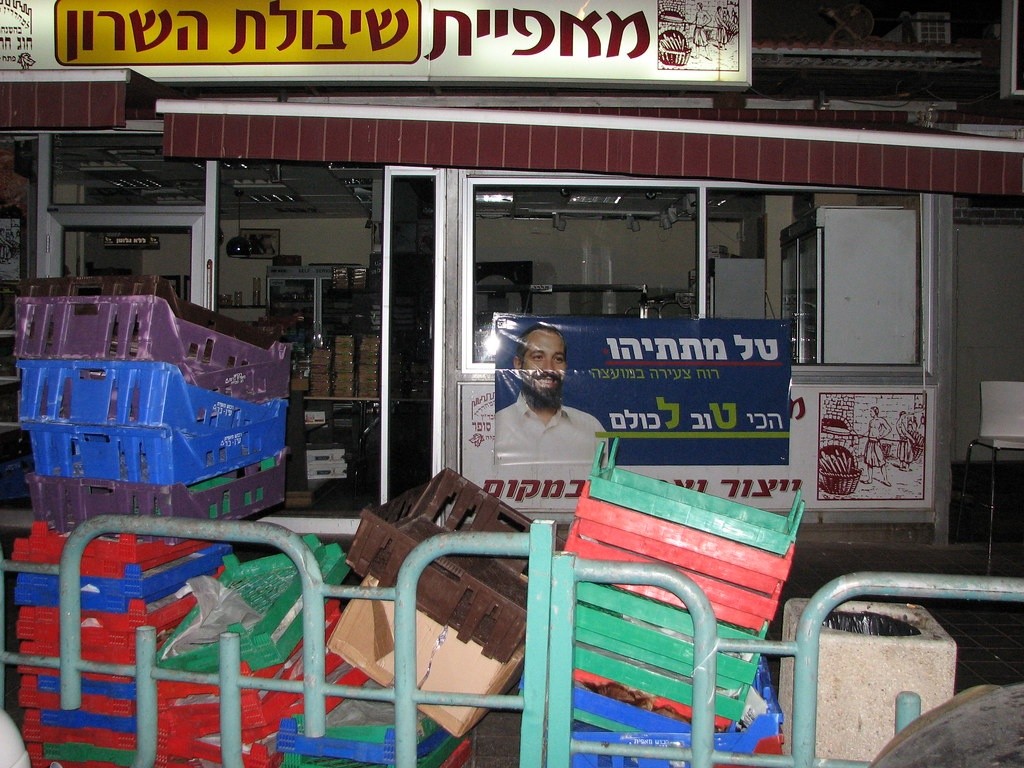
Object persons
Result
[495,324,609,462]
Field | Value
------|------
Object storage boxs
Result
[707,245,730,258]
[9,274,805,768]
[265,254,365,276]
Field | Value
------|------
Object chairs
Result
[955,381,1024,562]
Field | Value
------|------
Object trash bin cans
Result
[779,597,958,762]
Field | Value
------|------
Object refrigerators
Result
[689,257,766,318]
[781,206,920,363]
[266,262,367,356]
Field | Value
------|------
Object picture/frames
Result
[239,227,281,260]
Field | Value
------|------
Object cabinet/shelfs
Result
[267,278,369,348]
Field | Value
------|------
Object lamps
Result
[627,216,640,232]
[553,213,566,231]
[226,189,252,258]
[659,214,672,229]
[668,208,678,223]
